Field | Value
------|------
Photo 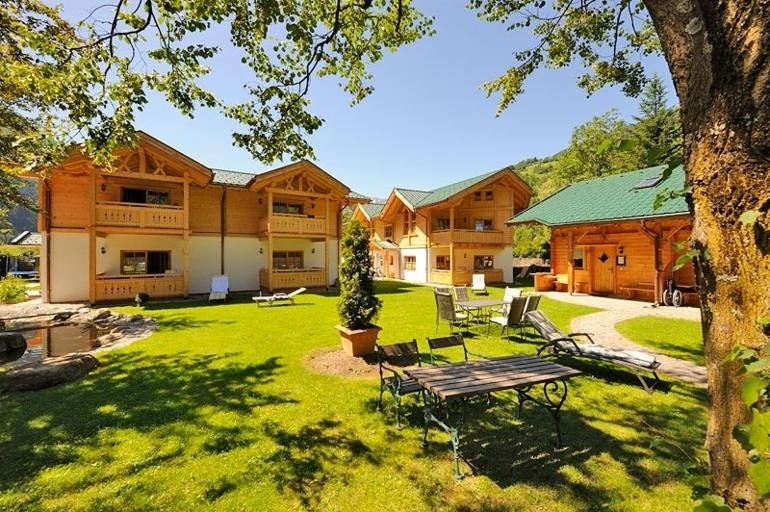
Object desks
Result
[402,353,584,480]
[451,300,511,333]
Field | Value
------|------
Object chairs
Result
[487,286,541,344]
[471,274,487,294]
[252,287,307,307]
[208,275,230,303]
[434,286,483,332]
[375,333,492,431]
[523,309,662,395]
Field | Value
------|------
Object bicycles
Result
[662,280,683,307]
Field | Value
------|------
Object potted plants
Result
[335,218,383,356]
